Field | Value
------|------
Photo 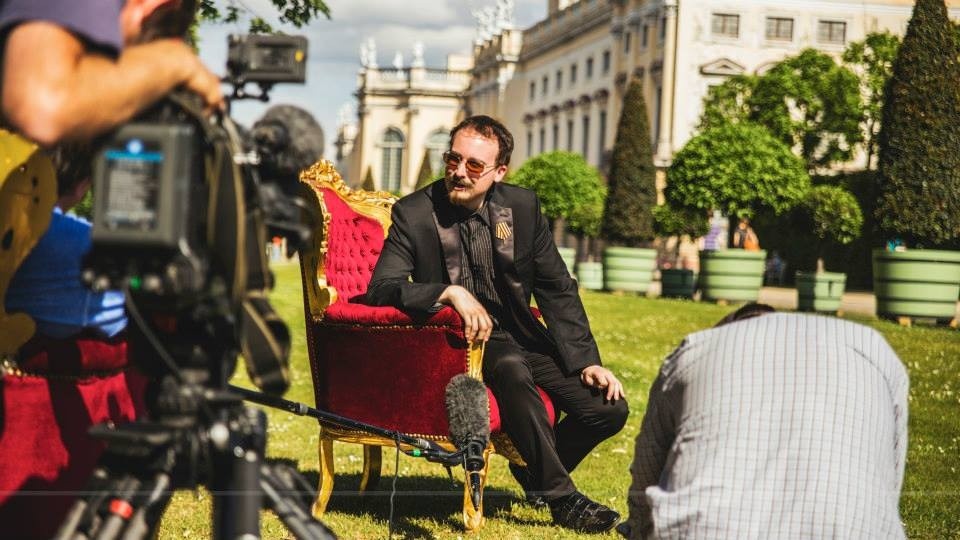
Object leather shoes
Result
[551,494,620,535]
[509,461,548,506]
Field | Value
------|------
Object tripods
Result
[50,342,340,540]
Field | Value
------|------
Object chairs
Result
[295,158,561,533]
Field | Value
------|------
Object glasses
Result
[442,150,498,173]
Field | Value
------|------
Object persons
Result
[626,300,910,540]
[0,0,225,375]
[368,115,629,534]
[733,218,781,287]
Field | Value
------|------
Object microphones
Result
[252,103,324,173]
[444,371,490,510]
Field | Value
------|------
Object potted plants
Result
[536,0,960,327]
